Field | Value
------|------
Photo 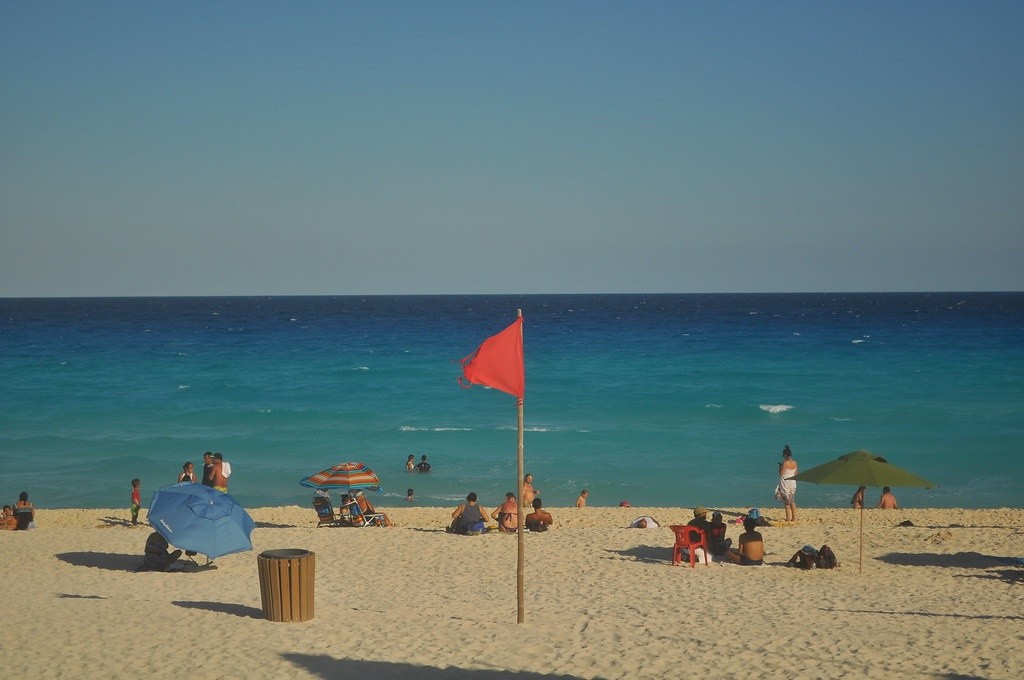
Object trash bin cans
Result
[256,548,316,623]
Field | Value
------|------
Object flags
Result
[458,317,523,399]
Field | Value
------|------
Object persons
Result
[850,485,866,509]
[340,488,396,527]
[405,454,431,472]
[177,451,229,495]
[491,492,553,532]
[131,478,141,525]
[630,515,660,528]
[876,487,896,509]
[0,491,35,530]
[405,488,415,502]
[521,473,540,508]
[779,445,798,522]
[576,490,589,508]
[451,492,489,535]
[144,531,182,569]
[687,506,763,565]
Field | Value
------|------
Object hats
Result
[209,453,222,460]
[693,505,710,515]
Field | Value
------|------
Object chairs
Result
[671,525,708,568]
[313,496,383,528]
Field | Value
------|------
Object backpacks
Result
[747,509,760,519]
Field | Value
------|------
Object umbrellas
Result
[299,461,384,525]
[145,480,257,568]
[783,450,932,574]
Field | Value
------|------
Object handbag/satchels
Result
[786,544,837,570]
